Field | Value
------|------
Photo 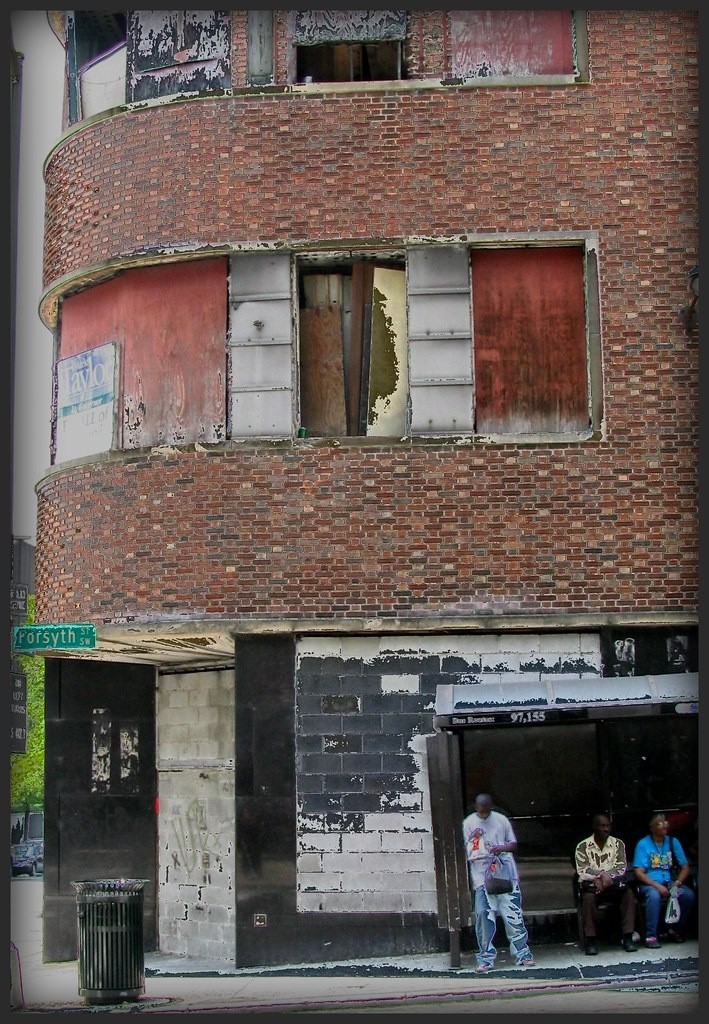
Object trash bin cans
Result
[69,875,151,1006]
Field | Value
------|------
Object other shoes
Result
[523,959,535,967]
[668,927,685,943]
[646,938,661,948]
[475,964,489,972]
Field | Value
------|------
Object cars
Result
[11,810,47,877]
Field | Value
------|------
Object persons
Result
[462,793,535,972]
[633,814,696,949]
[575,814,638,956]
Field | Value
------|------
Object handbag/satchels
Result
[669,837,694,885]
[484,855,514,895]
[583,876,627,891]
[665,887,683,924]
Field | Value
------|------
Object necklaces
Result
[651,834,665,866]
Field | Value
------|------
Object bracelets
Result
[676,879,682,884]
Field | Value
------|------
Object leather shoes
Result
[623,935,637,951]
[586,937,597,955]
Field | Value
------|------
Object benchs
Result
[514,869,606,950]
